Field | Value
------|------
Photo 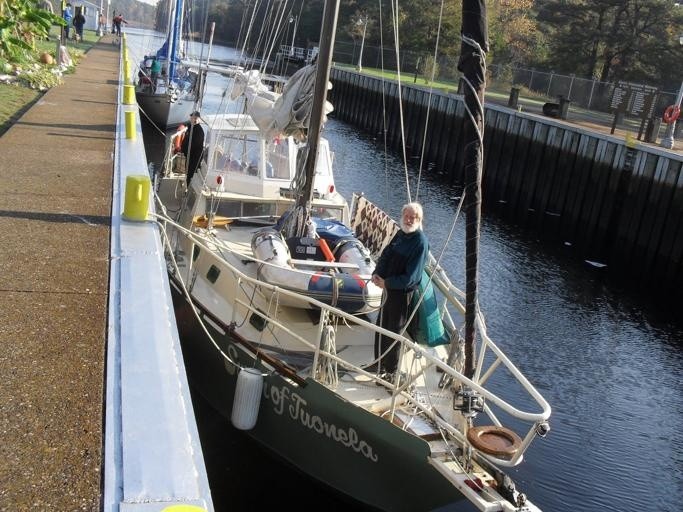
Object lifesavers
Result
[664,105,680,123]
[175,124,186,152]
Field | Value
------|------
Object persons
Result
[139,55,161,96]
[182,111,205,188]
[38,0,128,42]
[359,202,431,386]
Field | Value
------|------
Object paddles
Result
[192,215,280,227]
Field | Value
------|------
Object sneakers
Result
[361,362,396,386]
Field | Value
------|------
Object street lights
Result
[354,14,368,73]
[286,15,298,58]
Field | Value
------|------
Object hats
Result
[190,111,200,117]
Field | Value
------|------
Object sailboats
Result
[130,1,205,132]
[141,1,558,512]
